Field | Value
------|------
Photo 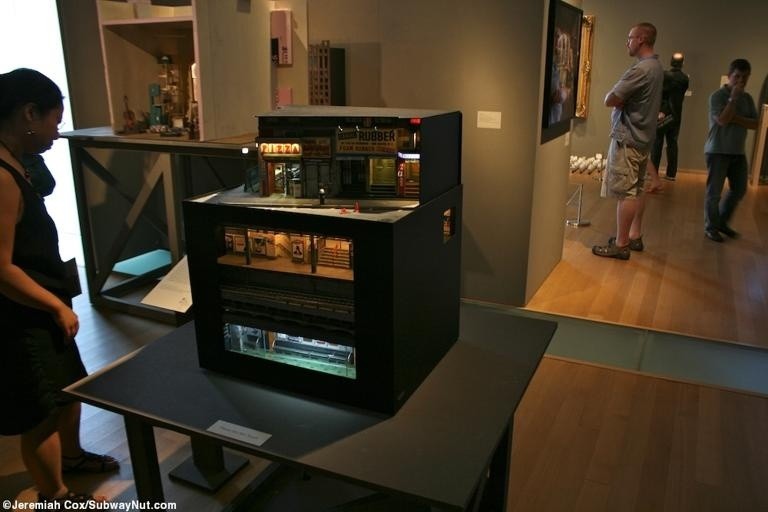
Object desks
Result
[61,302,558,511]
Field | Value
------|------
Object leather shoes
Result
[720,225,739,238]
[703,229,724,242]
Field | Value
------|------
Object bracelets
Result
[728,98,738,106]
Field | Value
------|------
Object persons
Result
[1,66,120,512]
[589,22,665,263]
[702,57,761,245]
[651,52,691,183]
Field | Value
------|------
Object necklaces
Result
[2,140,32,180]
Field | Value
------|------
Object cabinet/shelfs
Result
[58,126,260,327]
[57,0,271,141]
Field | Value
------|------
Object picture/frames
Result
[542,0,584,128]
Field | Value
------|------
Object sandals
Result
[35,490,110,512]
[591,241,630,259]
[61,448,121,474]
[610,235,646,251]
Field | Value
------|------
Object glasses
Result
[627,35,639,42]
